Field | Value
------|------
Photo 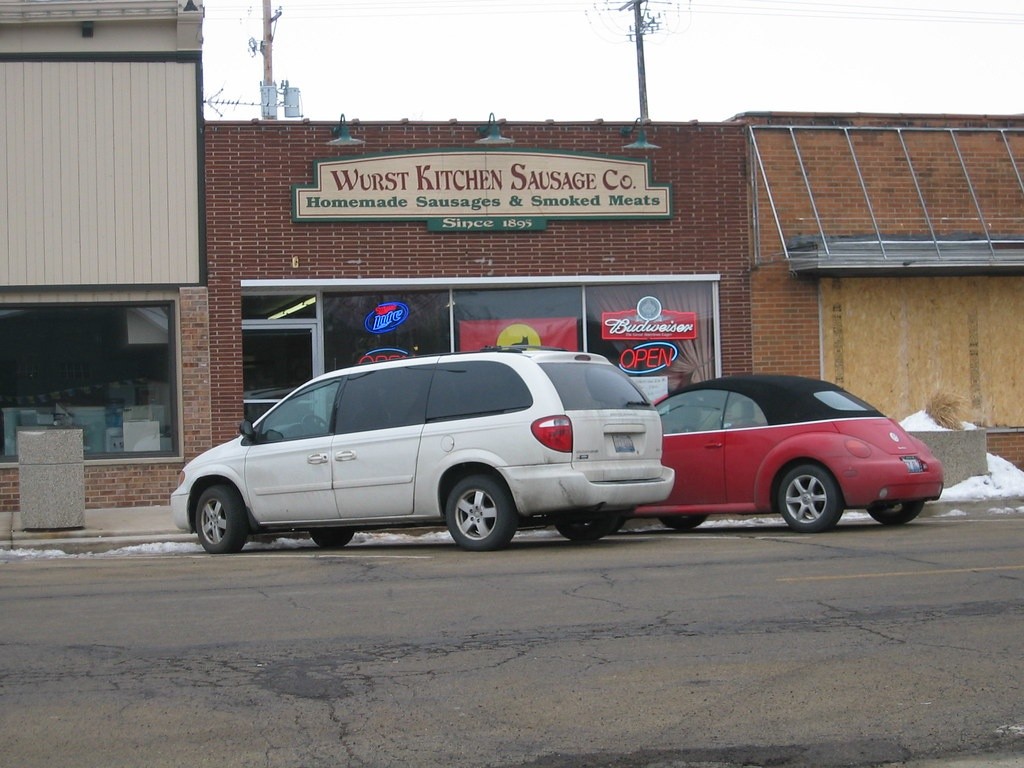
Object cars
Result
[629,371,944,534]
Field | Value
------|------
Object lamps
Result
[472,112,514,145]
[619,117,662,150]
[325,114,366,146]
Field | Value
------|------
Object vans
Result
[169,343,678,553]
